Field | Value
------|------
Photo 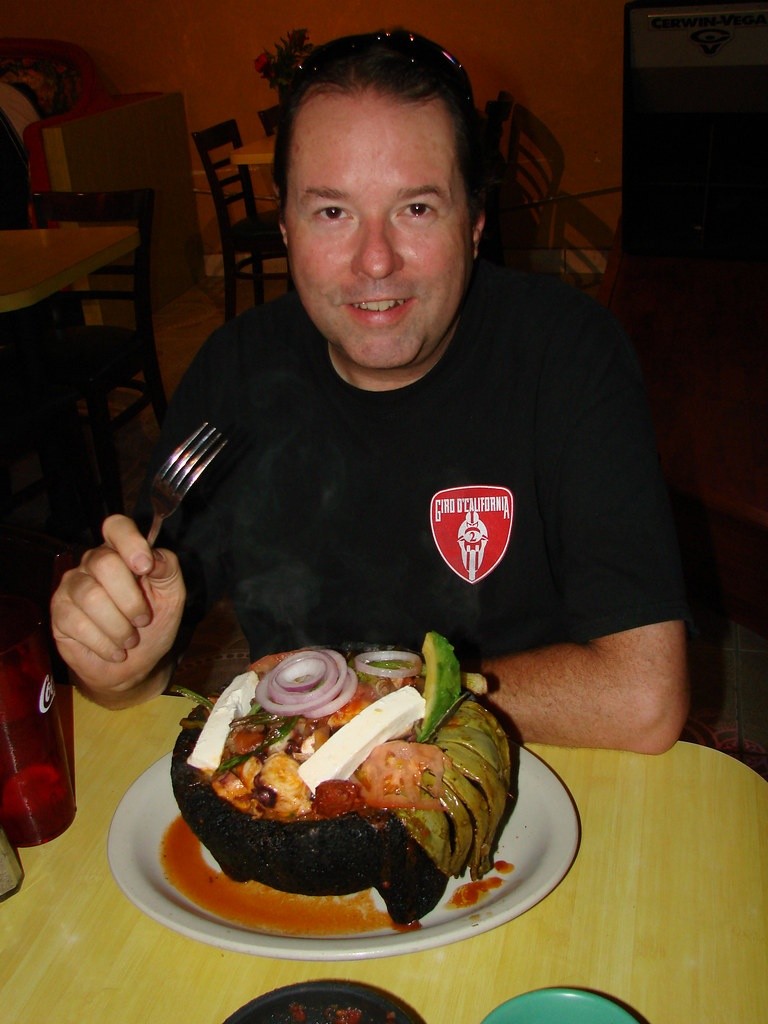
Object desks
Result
[611,255,768,641]
[0,684,768,1024]
[0,225,142,313]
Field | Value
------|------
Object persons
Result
[51,30,694,752]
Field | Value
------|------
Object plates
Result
[222,978,427,1024]
[106,750,582,962]
[481,987,640,1024]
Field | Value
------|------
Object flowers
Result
[252,26,316,90]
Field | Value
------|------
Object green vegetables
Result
[174,685,214,714]
[220,705,300,771]
[348,660,490,695]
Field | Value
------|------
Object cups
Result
[0,592,76,846]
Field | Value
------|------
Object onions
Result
[353,651,422,678]
[253,649,358,717]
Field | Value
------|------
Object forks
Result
[147,422,227,547]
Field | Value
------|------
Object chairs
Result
[31,188,167,516]
[192,119,291,324]
[481,92,512,262]
